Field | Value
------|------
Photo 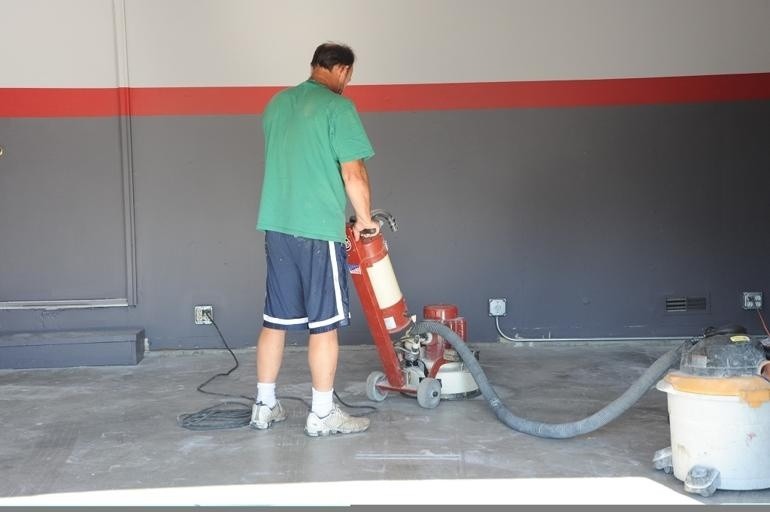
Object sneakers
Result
[305,405,369,437]
[250,401,287,429]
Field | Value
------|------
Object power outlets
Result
[743,291,763,311]
[194,305,214,324]
[489,298,507,318]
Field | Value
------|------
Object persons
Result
[247,41,382,439]
